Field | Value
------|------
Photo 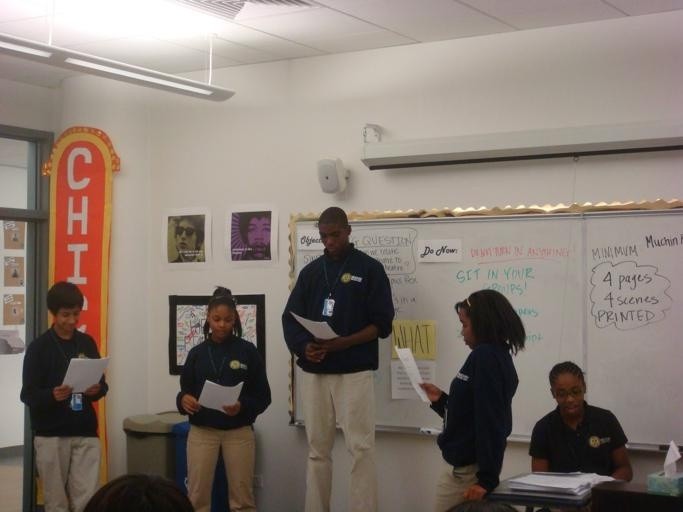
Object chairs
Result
[443,499,520,511]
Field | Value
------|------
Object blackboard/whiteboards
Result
[294,208,683,454]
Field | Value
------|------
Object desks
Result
[485,474,611,511]
[588,470,682,511]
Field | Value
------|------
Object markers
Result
[416,428,443,436]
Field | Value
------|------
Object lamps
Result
[0,0,237,103]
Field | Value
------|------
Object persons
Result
[176,286,271,512]
[238,211,271,260]
[529,361,633,511]
[418,288,526,512]
[171,214,205,262]
[20,281,108,512]
[84,473,196,512]
[282,207,395,512]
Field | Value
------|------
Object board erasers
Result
[660,445,683,451]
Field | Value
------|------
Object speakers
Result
[316,156,351,193]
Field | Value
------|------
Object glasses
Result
[175,226,196,236]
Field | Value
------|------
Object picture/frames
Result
[168,293,267,378]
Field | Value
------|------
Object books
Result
[508,472,615,502]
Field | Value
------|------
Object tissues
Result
[647,440,683,497]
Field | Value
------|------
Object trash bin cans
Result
[123,411,228,512]
